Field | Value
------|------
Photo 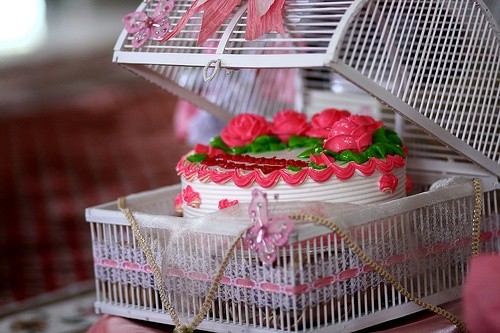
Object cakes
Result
[173,109,415,252]
